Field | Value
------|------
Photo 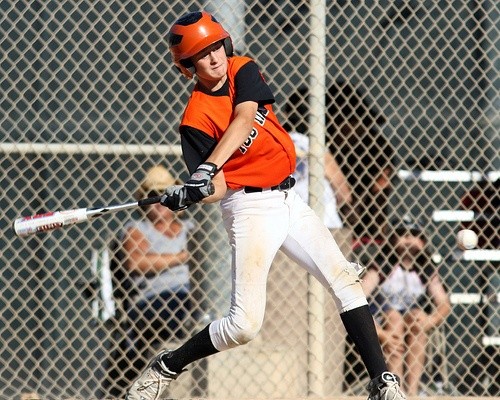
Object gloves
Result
[182,162,218,204]
[160,185,192,212]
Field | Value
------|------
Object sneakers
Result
[365,371,408,400]
[124,350,187,400]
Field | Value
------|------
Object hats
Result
[136,165,175,202]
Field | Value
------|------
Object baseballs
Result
[455,229,480,251]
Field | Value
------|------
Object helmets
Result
[387,215,424,230]
[169,12,233,79]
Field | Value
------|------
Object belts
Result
[245,176,295,193]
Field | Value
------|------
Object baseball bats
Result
[12,182,215,237]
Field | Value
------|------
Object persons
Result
[282,81,450,400]
[126,12,415,400]
[102,166,198,400]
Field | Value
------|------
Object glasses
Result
[147,188,160,198]
[395,228,421,236]
